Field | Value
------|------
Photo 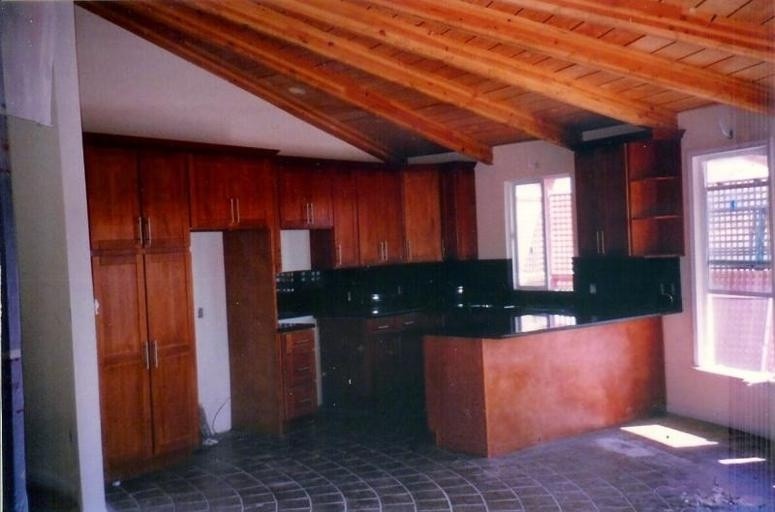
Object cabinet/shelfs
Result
[82,131,478,485]
[571,128,686,259]
